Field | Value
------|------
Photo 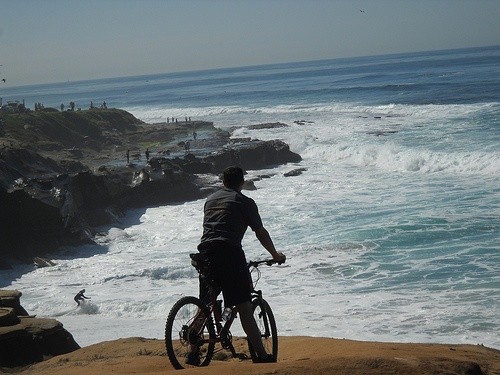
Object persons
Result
[35,101,197,162]
[184,166,286,364]
[74,289,91,305]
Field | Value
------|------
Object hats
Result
[222,165,244,186]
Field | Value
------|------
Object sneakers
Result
[253,354,276,363]
[185,353,201,366]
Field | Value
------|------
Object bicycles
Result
[164,259,279,370]
